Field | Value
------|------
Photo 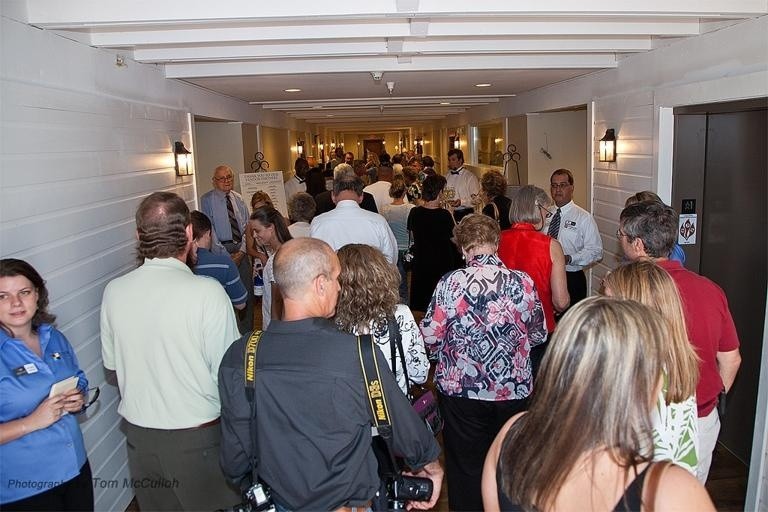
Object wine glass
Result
[439,186,455,210]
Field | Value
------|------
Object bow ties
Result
[299,179,306,184]
[451,170,459,175]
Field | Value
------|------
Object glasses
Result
[215,175,234,182]
[615,228,630,242]
[68,387,100,414]
[551,182,566,188]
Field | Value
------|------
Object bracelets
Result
[19,416,27,436]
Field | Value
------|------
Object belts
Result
[220,240,234,245]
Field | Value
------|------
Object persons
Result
[0,258,94,512]
[481,295,718,512]
[218,237,444,512]
[602,261,698,482]
[619,190,686,267]
[330,243,431,511]
[419,213,548,511]
[188,146,602,335]
[617,200,742,486]
[100,191,241,510]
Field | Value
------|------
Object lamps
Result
[598,128,616,162]
[175,142,194,177]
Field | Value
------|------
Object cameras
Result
[386,475,433,510]
[229,484,277,512]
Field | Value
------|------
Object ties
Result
[223,192,243,244]
[547,207,561,240]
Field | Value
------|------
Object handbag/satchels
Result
[252,258,266,296]
[403,244,414,272]
[406,386,445,438]
